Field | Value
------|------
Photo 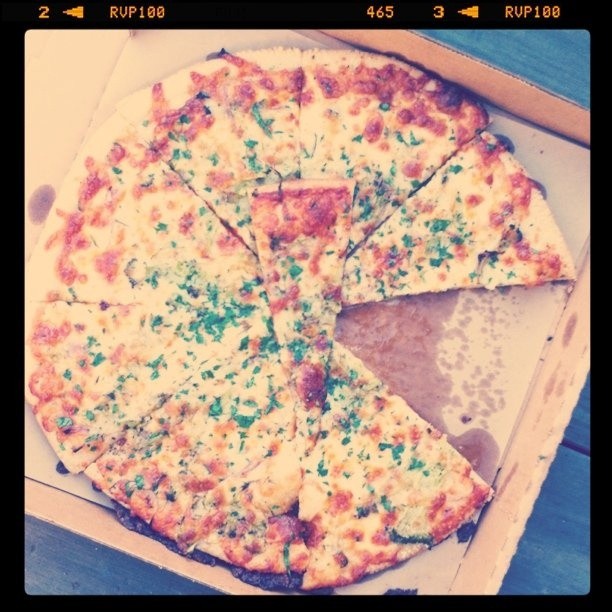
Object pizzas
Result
[25,48,579,590]
